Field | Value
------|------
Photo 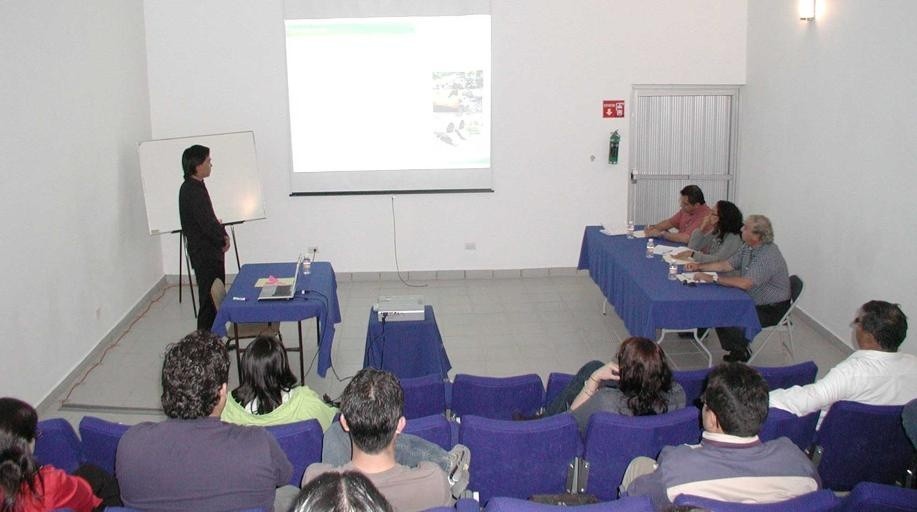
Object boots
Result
[513,410,543,421]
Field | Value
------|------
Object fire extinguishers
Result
[608,129,620,164]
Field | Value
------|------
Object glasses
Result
[681,279,696,287]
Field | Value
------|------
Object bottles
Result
[302,254,312,275]
[646,238,655,259]
[626,220,634,240]
[668,259,678,280]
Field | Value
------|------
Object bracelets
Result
[690,251,694,258]
[713,272,719,284]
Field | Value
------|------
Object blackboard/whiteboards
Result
[137,130,267,236]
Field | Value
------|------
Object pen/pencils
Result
[648,224,649,229]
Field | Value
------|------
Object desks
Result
[577,226,762,369]
[212,262,341,386]
[363,305,452,377]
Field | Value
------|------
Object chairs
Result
[746,274,804,366]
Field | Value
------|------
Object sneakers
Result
[679,331,709,338]
[450,444,471,498]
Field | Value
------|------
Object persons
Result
[179,144,237,351]
[680,214,792,361]
[2,329,481,512]
[512,302,917,512]
[674,200,746,264]
[645,185,714,242]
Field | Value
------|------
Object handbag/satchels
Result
[529,493,598,505]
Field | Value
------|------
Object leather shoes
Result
[723,347,752,362]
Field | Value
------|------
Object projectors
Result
[378,294,425,322]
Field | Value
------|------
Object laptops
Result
[258,255,301,301]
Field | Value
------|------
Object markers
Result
[232,297,249,301]
